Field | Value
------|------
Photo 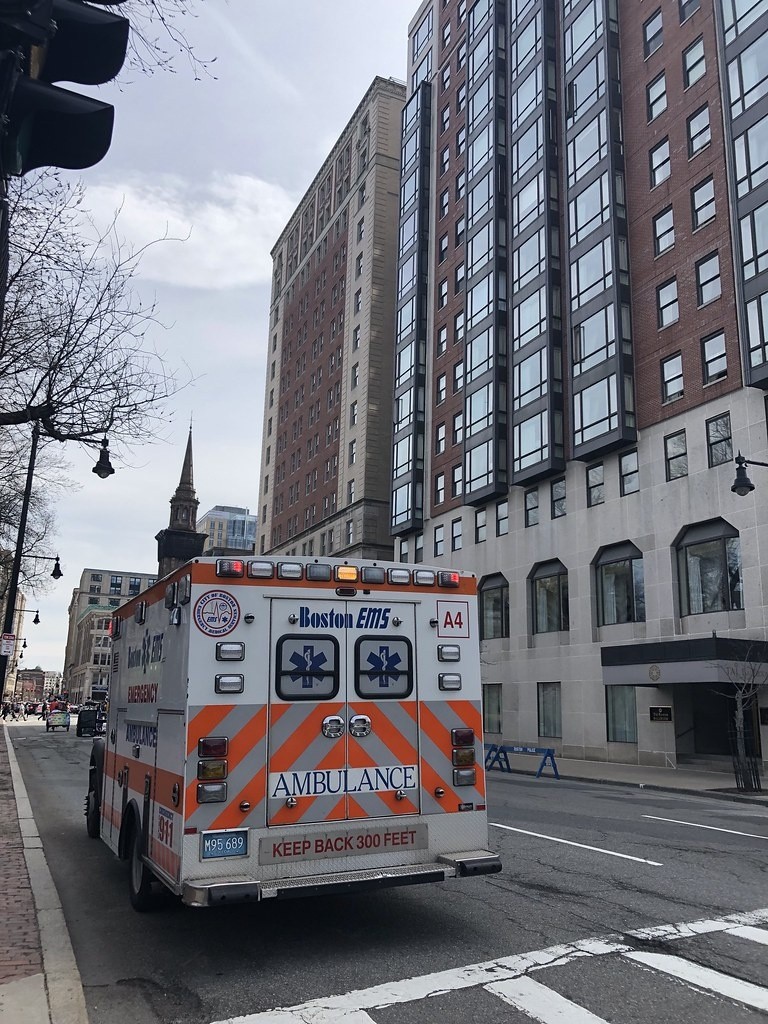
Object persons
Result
[0,686,112,721]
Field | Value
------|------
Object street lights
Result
[730,449,768,497]
[1,421,116,725]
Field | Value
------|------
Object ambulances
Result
[88,552,499,918]
